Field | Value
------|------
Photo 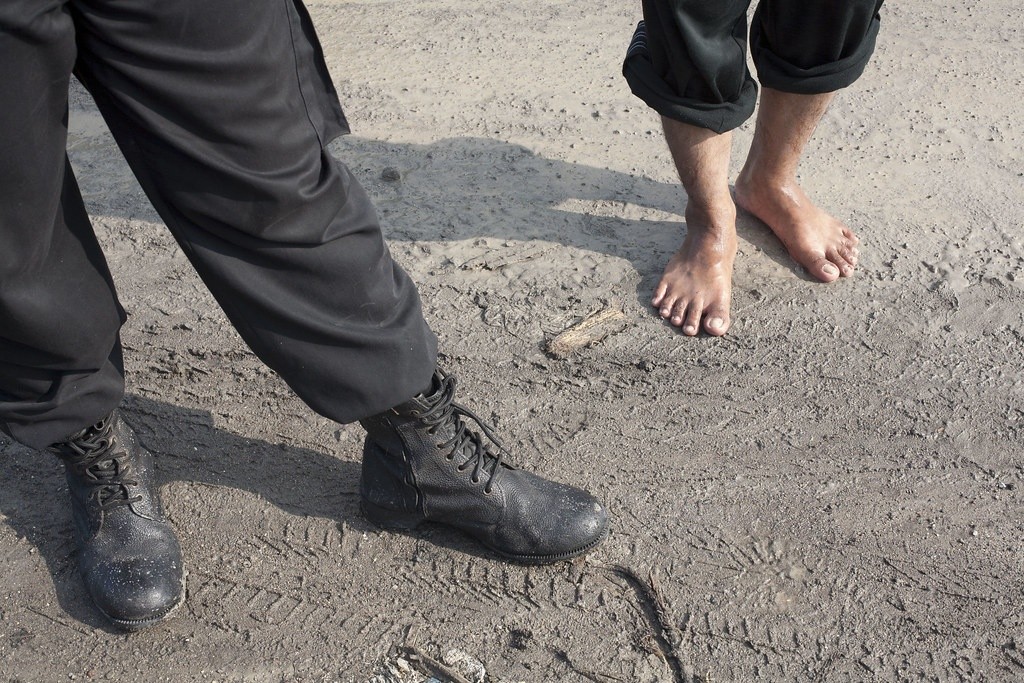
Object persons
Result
[0,0,612,630]
[623,0,886,336]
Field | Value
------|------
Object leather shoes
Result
[357,370,606,567]
[51,407,187,630]
